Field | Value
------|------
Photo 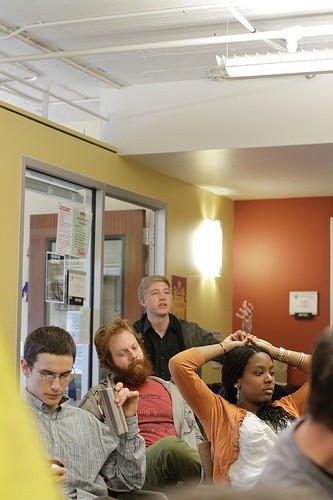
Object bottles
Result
[98,388,129,436]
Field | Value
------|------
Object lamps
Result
[216,27,333,79]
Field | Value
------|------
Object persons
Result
[78,318,205,492]
[168,330,312,491]
[20,326,146,500]
[133,275,254,380]
[257,325,333,500]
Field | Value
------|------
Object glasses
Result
[26,361,76,382]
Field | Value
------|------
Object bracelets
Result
[277,346,285,361]
[295,352,305,370]
[220,344,226,354]
[286,350,290,363]
